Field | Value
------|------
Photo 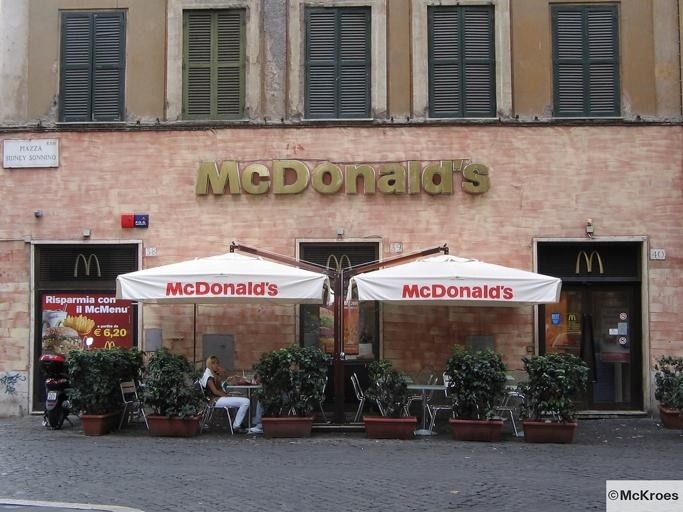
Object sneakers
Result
[245,424,262,432]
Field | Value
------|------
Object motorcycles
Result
[38,337,93,430]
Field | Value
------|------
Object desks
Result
[227,378,262,435]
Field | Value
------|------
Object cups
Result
[79,331,89,350]
[41,309,65,337]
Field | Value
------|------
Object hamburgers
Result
[552,331,581,355]
[41,327,82,356]
[319,308,348,346]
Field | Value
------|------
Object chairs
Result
[350,366,522,436]
[118,378,150,432]
[200,379,234,435]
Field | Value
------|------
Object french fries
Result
[63,314,95,335]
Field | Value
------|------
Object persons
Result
[201,355,251,435]
[248,391,288,434]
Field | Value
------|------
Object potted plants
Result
[523,351,588,444]
[67,345,140,437]
[140,346,199,437]
[261,344,322,436]
[654,354,683,429]
